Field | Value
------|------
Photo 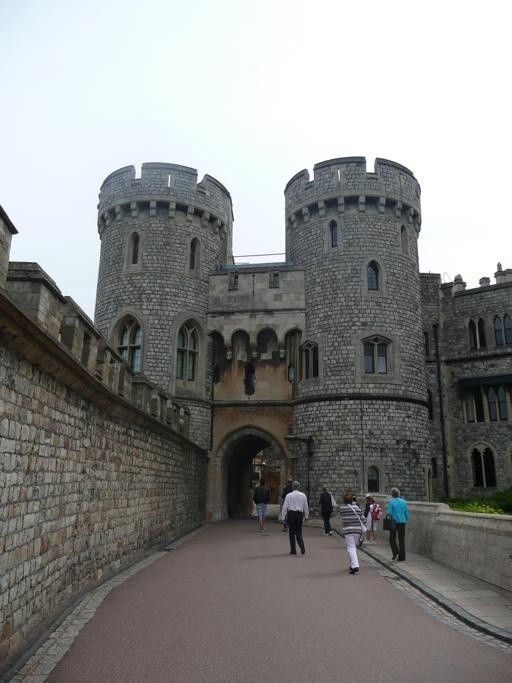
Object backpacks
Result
[369,503,383,520]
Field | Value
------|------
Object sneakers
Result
[328,530,332,536]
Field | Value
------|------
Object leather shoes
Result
[350,566,359,573]
[350,566,353,569]
[392,550,399,558]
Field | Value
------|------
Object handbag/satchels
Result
[383,513,396,529]
[328,491,337,507]
[361,522,367,533]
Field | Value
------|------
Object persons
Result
[364,493,374,536]
[340,493,366,574]
[386,487,409,561]
[319,485,333,535]
[282,478,292,530]
[364,497,378,544]
[253,478,269,531]
[279,481,308,554]
[352,495,363,545]
